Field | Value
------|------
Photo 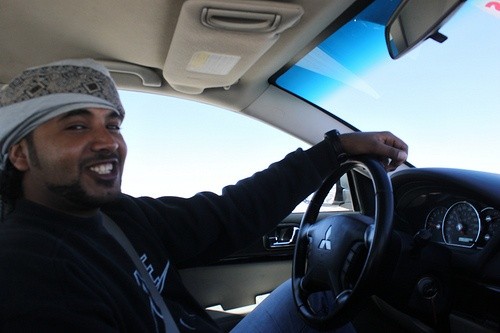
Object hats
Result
[0,57,124,172]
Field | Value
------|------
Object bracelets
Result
[324,129,347,160]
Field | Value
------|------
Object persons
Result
[0,57,410,333]
